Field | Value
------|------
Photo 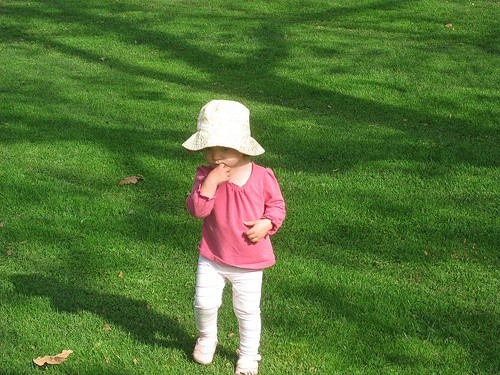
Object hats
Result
[181,100,265,156]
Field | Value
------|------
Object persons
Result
[180,99,286,375]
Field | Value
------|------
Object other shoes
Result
[235,354,261,375]
[193,338,216,364]
